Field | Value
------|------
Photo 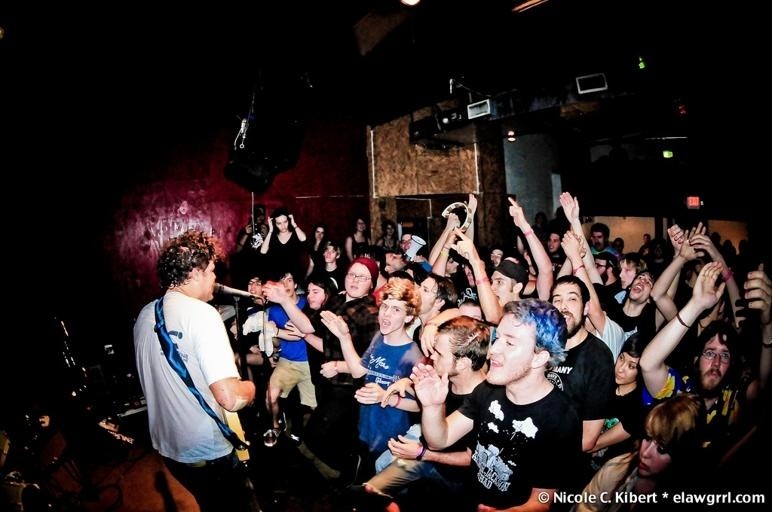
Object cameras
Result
[251,221,261,234]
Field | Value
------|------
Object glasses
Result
[700,349,732,364]
[346,272,372,283]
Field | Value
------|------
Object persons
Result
[136,229,261,511]
[216,191,772,512]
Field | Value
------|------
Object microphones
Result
[214,283,257,299]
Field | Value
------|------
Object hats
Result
[493,260,530,283]
[348,257,379,276]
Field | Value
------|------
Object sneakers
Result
[263,412,289,447]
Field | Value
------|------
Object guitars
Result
[221,404,251,463]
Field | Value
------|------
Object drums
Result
[0,471,46,511]
[0,429,11,468]
